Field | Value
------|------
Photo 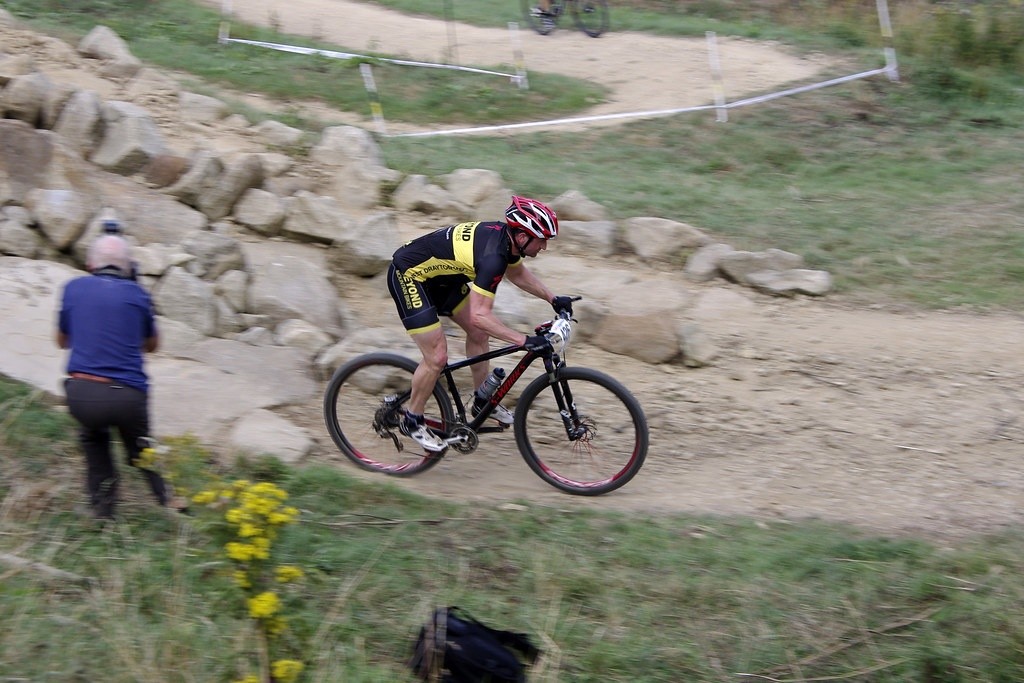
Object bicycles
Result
[324,294,650,497]
[521,0,608,38]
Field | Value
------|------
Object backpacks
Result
[412,606,540,683]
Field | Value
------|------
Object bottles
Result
[478,368,506,399]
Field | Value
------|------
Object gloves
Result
[552,296,573,317]
[524,335,555,357]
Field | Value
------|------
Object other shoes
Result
[164,495,190,512]
[84,512,109,532]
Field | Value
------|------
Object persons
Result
[387,195,573,452]
[538,0,556,16]
[56,235,190,529]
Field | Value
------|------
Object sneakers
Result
[471,400,515,424]
[398,411,446,452]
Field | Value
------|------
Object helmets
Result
[505,195,559,239]
[87,235,130,274]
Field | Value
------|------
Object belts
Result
[72,372,112,383]
